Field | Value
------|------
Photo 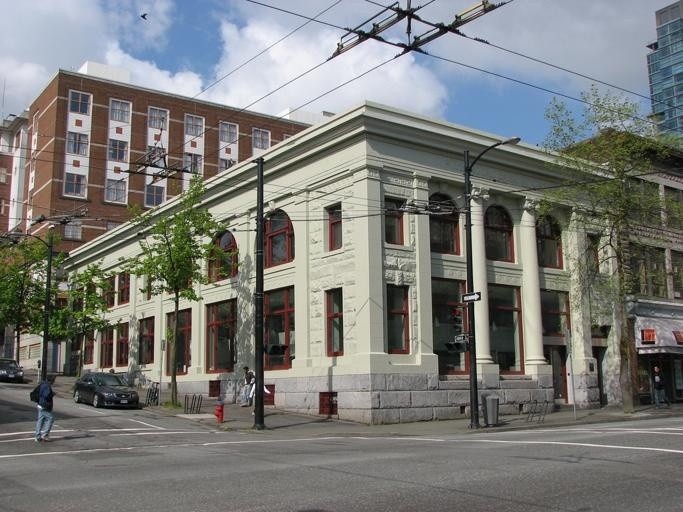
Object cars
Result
[0,357,24,383]
[70,372,139,410]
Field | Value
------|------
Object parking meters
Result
[36,359,41,382]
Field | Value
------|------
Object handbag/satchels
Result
[31,385,40,402]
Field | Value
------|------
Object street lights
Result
[460,132,521,431]
[5,230,54,383]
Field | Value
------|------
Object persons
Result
[651,366,671,409]
[33,374,59,443]
[250,377,271,416]
[239,366,253,407]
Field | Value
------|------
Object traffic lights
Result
[443,340,468,354]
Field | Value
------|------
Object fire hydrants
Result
[212,396,225,424]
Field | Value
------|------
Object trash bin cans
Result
[482,393,499,427]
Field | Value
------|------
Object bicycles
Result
[144,378,159,408]
